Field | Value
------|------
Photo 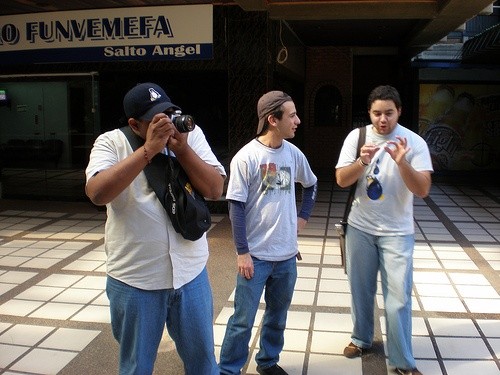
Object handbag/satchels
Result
[334,220,347,275]
[144,149,212,242]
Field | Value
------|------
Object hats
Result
[124,82,182,122]
[256,90,290,135]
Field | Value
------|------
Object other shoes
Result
[395,367,423,375]
[343,342,369,358]
[256,364,289,375]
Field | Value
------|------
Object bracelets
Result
[356,157,371,167]
[143,144,151,164]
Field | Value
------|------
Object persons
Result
[335,86,434,375]
[84,84,227,375]
[219,91,319,375]
